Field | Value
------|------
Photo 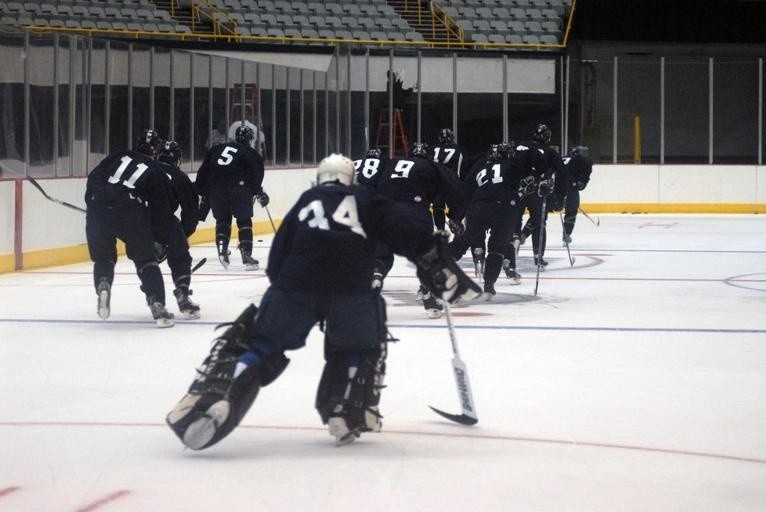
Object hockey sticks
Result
[189,258,208,274]
[534,195,547,295]
[578,207,599,227]
[26,176,87,212]
[560,213,575,266]
[428,299,477,425]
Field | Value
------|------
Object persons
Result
[386,70,413,135]
[356,124,592,319]
[196,126,269,271]
[165,153,483,450]
[86,129,201,328]
[85,111,267,160]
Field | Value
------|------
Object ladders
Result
[376,109,410,161]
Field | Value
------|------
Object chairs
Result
[1,0,580,50]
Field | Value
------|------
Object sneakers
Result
[563,233,572,243]
[534,253,548,267]
[173,287,201,313]
[473,255,522,295]
[327,407,359,446]
[96,281,110,319]
[420,286,444,312]
[151,301,175,320]
[217,239,231,265]
[183,400,230,450]
[239,246,259,264]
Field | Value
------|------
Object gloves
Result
[538,178,555,197]
[410,240,459,302]
[255,187,270,207]
[449,219,465,237]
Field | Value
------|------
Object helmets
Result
[569,145,591,157]
[366,147,390,160]
[316,153,356,186]
[438,128,456,142]
[530,123,552,148]
[235,126,254,145]
[409,142,431,158]
[156,140,183,162]
[136,129,164,156]
[488,141,516,162]
